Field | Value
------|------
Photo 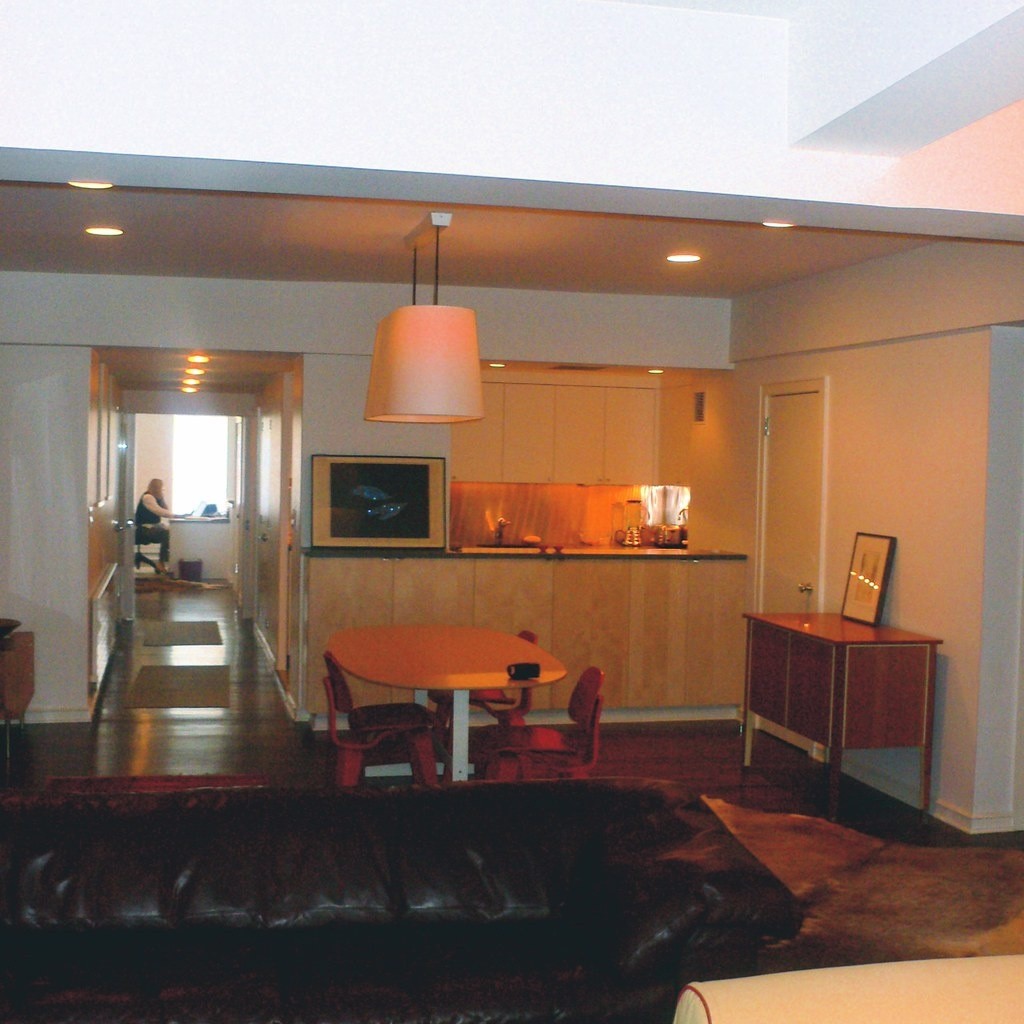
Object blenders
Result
[622,501,647,547]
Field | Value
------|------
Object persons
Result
[134,479,191,574]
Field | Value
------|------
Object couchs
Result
[1,781,806,1024]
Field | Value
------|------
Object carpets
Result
[123,665,231,707]
[144,620,223,645]
[44,776,276,791]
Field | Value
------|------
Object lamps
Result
[364,212,486,424]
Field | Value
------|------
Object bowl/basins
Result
[0,618,21,638]
[579,533,611,546]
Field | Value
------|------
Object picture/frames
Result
[310,454,447,550]
[841,532,897,626]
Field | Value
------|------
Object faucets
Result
[495,518,511,544]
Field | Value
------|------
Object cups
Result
[655,526,681,544]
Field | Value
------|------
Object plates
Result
[654,542,687,548]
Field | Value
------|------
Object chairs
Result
[673,955,1024,1024]
[323,630,604,788]
[133,534,161,574]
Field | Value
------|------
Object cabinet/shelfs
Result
[741,612,944,823]
[451,382,655,486]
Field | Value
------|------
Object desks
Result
[0,631,34,760]
[168,517,230,580]
[327,624,567,782]
